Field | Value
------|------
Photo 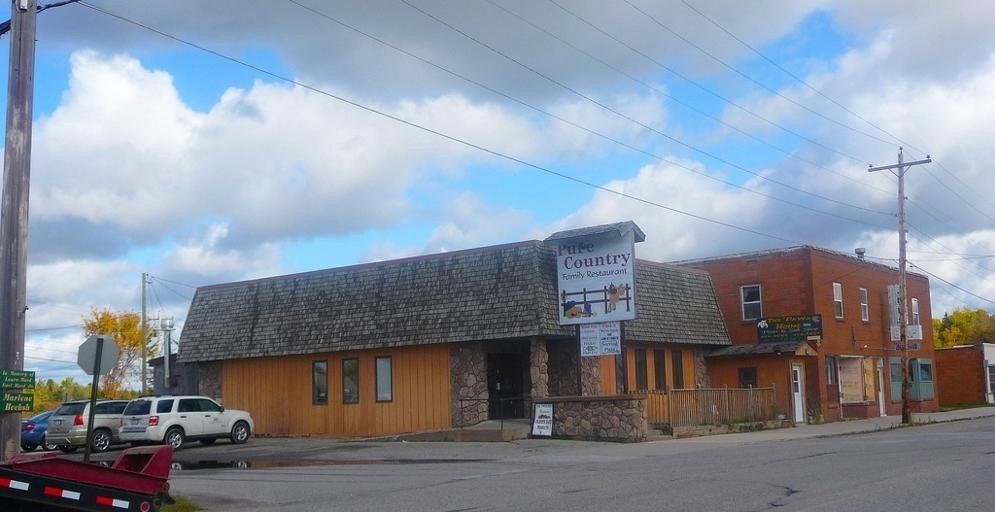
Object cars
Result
[21,394,254,453]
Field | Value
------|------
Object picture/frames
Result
[375,355,393,402]
[343,357,359,404]
[312,360,328,405]
